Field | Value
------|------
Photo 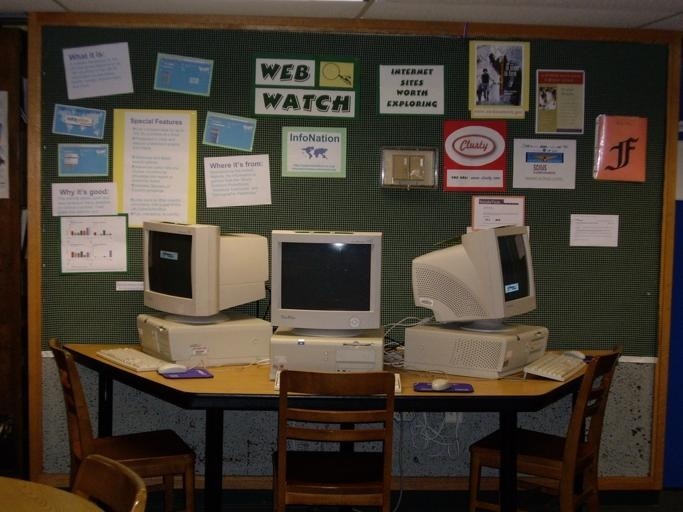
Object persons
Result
[479,68,493,100]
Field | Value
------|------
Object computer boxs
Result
[404,323,549,379]
[137,312,273,368]
[269,326,385,380]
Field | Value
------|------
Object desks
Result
[62,341,601,512]
[0,476,104,511]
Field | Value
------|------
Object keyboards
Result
[96,347,168,372]
[523,352,586,382]
[273,371,402,393]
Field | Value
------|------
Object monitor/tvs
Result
[144,221,269,324]
[271,230,382,338]
[412,226,537,333]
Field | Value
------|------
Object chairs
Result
[468,350,622,510]
[272,367,395,510]
[69,455,147,510]
[49,337,195,508]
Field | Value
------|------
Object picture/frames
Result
[379,145,437,188]
[282,127,348,179]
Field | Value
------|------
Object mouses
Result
[158,364,187,374]
[432,378,452,391]
[564,350,586,359]
